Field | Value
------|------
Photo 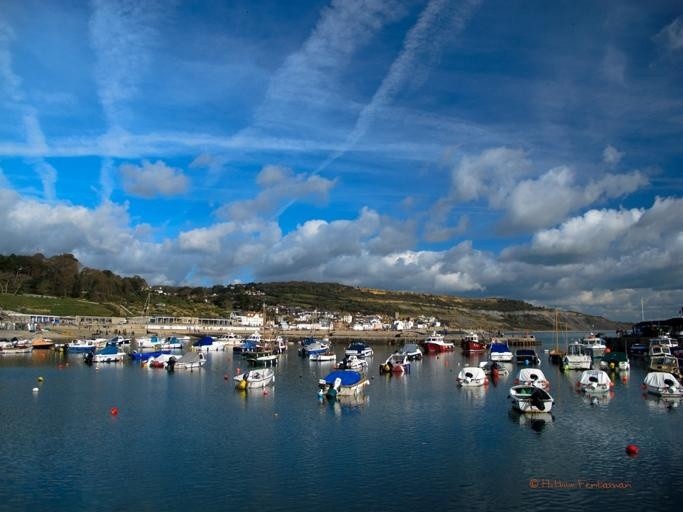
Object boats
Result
[316,394,369,417]
[509,407,556,429]
[503,381,554,413]
[313,366,370,397]
[639,388,680,411]
[638,369,683,400]
